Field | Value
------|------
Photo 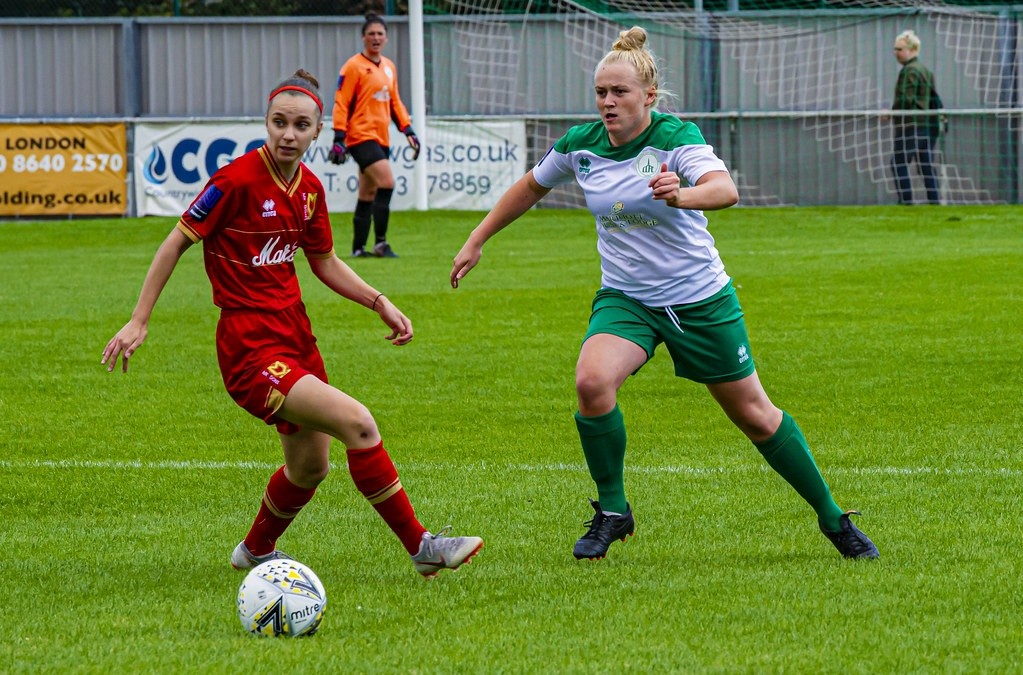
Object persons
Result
[450,26,879,564]
[890,31,946,205]
[99,69,484,578]
[328,11,421,258]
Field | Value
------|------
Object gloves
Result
[406,126,420,160]
[328,130,346,165]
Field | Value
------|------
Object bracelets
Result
[373,293,384,311]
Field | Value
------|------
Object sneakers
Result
[411,525,484,578]
[573,497,634,561]
[818,510,879,561]
[231,540,294,570]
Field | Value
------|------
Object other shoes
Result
[374,242,398,258]
[353,248,374,257]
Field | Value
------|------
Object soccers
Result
[239,556,328,638]
